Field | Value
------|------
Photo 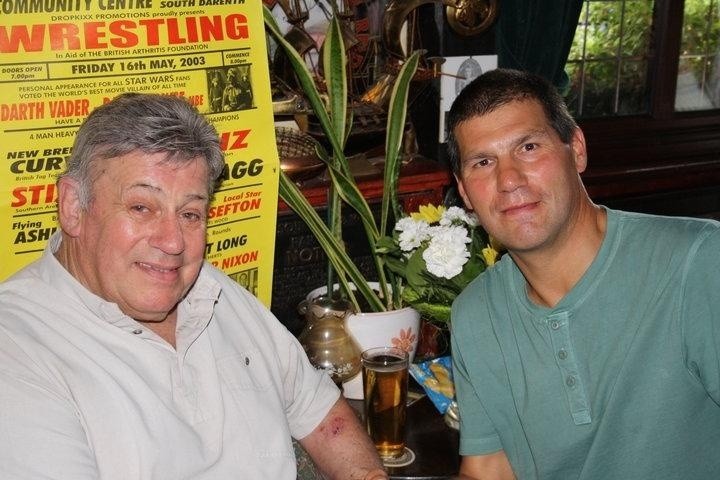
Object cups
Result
[358,346,410,461]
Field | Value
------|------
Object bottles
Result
[296,296,363,386]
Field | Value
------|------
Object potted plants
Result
[263,0,429,400]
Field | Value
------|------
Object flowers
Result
[376,198,502,326]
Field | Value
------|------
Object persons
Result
[445,67,719,479]
[207,66,253,111]
[3,91,389,479]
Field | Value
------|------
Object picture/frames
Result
[438,52,499,145]
